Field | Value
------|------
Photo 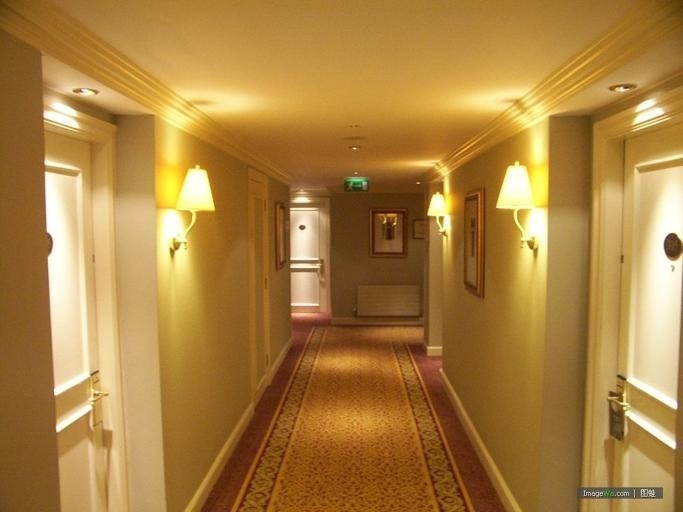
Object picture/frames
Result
[461,183,485,300]
[368,206,424,260]
[274,201,287,271]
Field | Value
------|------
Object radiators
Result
[354,284,421,317]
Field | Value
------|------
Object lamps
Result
[495,161,539,252]
[426,191,448,238]
[169,163,215,253]
